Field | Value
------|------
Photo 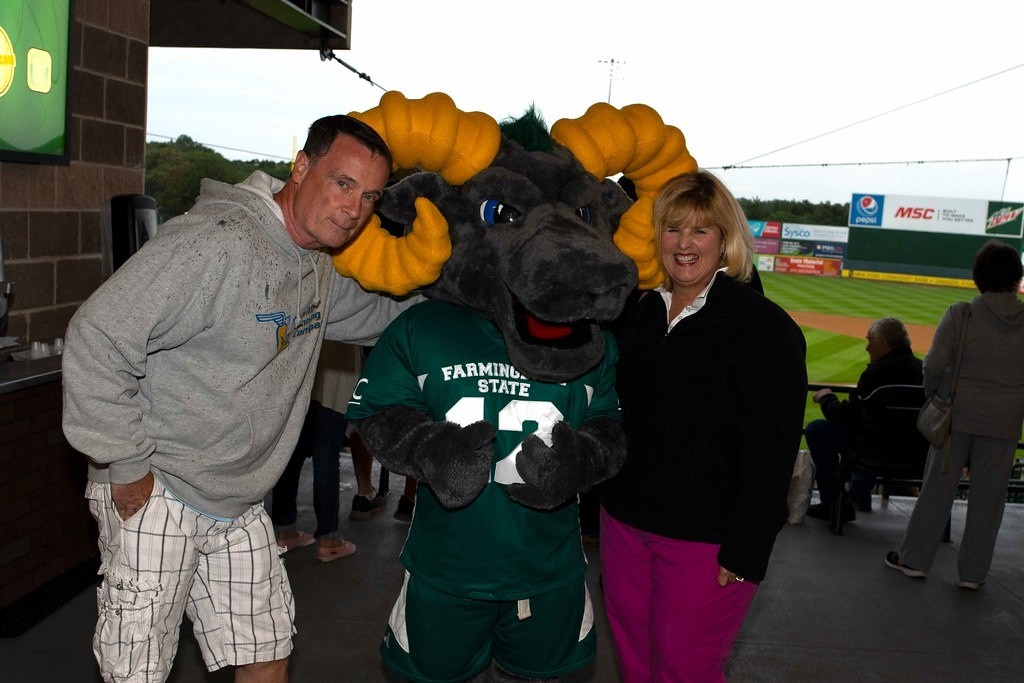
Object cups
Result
[31,337,63,360]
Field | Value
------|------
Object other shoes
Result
[806,503,856,521]
[852,493,872,512]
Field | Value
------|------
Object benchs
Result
[829,385,1024,535]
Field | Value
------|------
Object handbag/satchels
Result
[916,393,951,448]
[788,451,816,523]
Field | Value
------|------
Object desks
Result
[0,344,102,588]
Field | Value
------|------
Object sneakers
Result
[277,531,315,551]
[394,495,414,522]
[885,551,928,577]
[350,494,386,520]
[318,538,356,562]
[959,579,987,589]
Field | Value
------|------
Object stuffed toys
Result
[324,92,700,678]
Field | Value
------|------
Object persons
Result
[595,169,808,683]
[60,114,428,683]
[883,240,1023,590]
[803,317,927,527]
